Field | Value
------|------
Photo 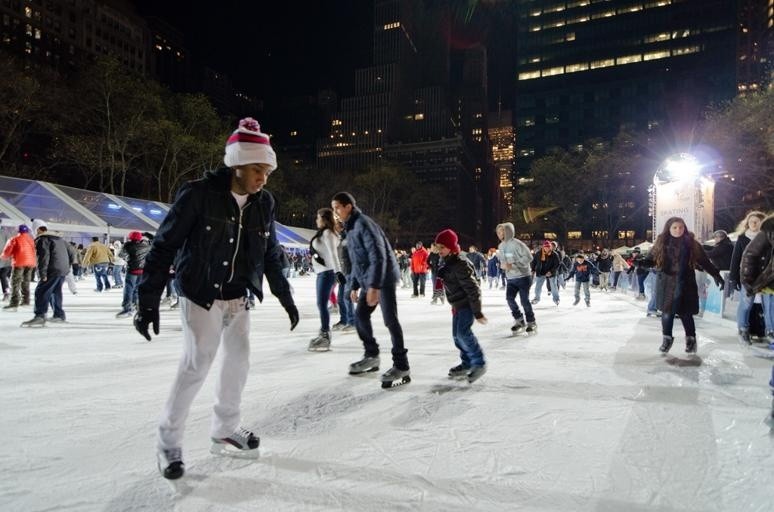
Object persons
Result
[1,217,156,328]
[624,209,773,356]
[414,229,489,384]
[292,189,413,391]
[488,221,652,336]
[131,119,299,481]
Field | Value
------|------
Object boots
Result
[526,319,539,337]
[112,285,121,288]
[116,284,124,287]
[327,304,339,314]
[347,348,382,376]
[659,333,672,353]
[379,354,411,389]
[685,333,697,354]
[307,328,332,353]
[511,314,526,336]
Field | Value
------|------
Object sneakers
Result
[530,297,540,305]
[737,328,752,346]
[211,426,260,460]
[330,322,346,332]
[646,310,662,318]
[19,314,46,328]
[155,444,187,480]
[103,287,111,292]
[765,331,774,348]
[448,363,469,380]
[92,287,102,293]
[439,296,445,305]
[46,313,66,323]
[572,299,581,306]
[170,297,181,310]
[19,302,31,307]
[2,304,18,313]
[553,298,561,306]
[71,292,81,297]
[466,365,487,385]
[116,305,133,318]
[342,324,358,335]
[585,299,591,308]
[430,297,438,305]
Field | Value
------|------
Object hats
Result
[17,224,29,233]
[433,229,462,254]
[31,217,48,235]
[127,231,143,241]
[542,239,552,247]
[223,117,278,170]
[632,247,641,254]
[575,249,585,259]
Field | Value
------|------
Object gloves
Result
[730,278,742,292]
[130,304,163,344]
[278,291,303,333]
[334,272,347,286]
[713,272,725,292]
